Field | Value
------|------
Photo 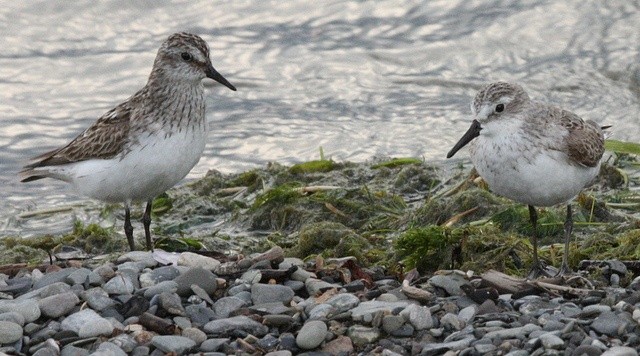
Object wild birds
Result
[446,80,615,278]
[14,30,239,255]
